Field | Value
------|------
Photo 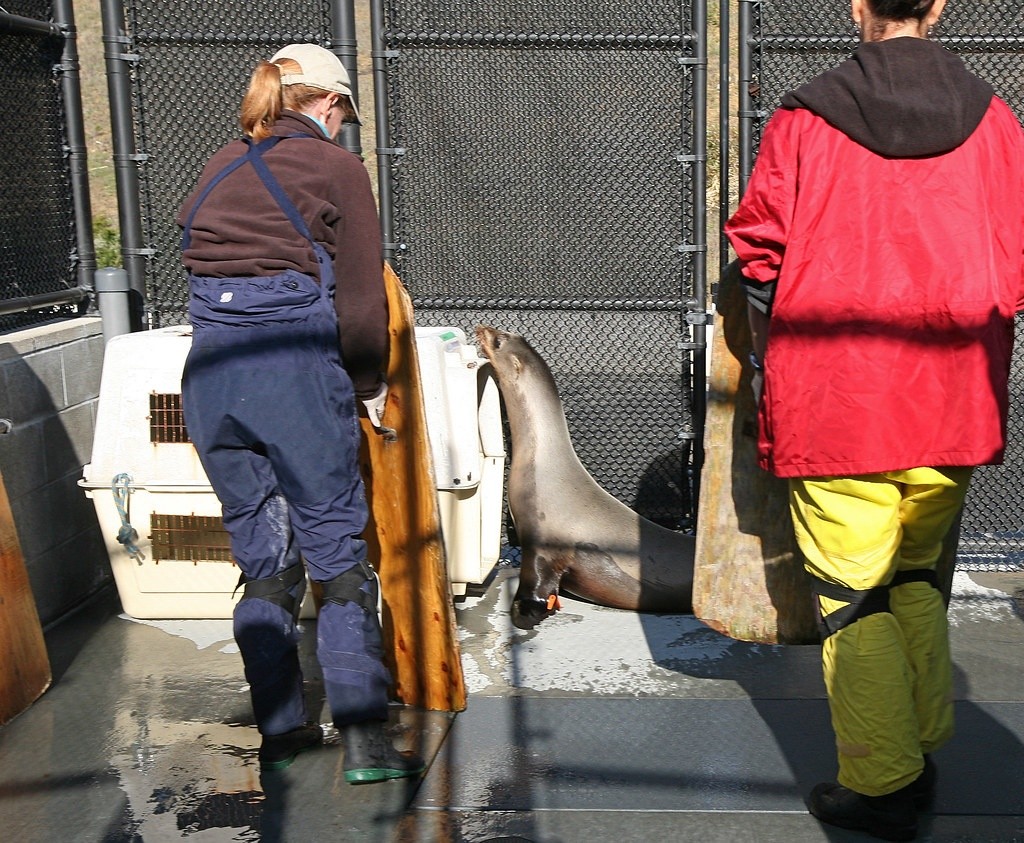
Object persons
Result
[175,43,425,782]
[723,0,1024,843]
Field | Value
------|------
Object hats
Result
[269,44,366,126]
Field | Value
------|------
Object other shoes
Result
[342,736,425,782]
[259,727,325,770]
[806,751,941,843]
